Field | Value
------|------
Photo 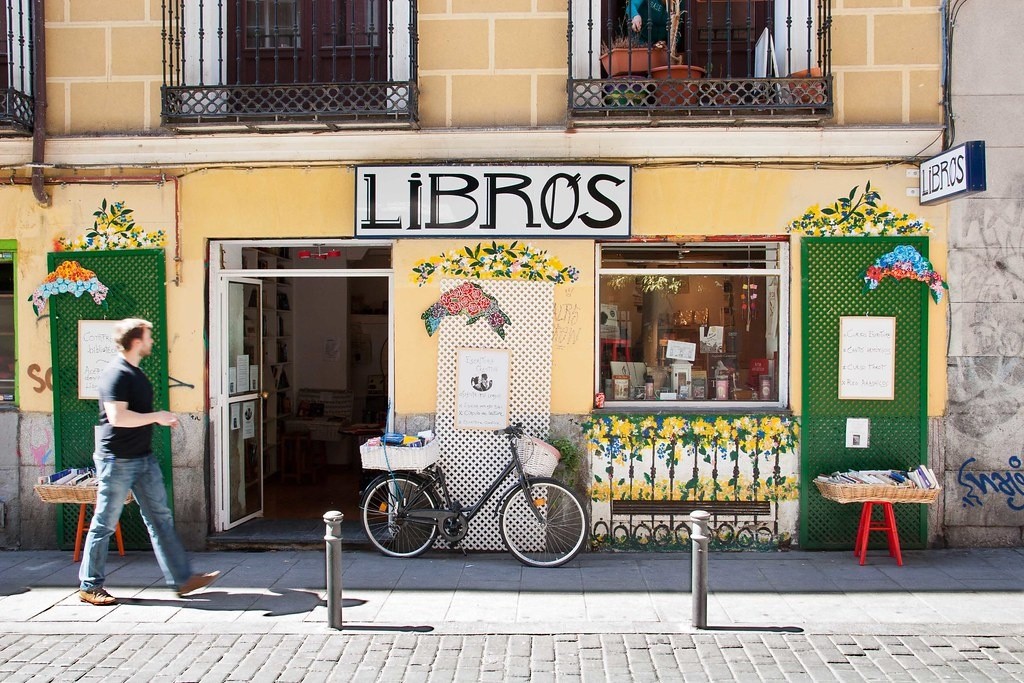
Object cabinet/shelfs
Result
[658,324,709,372]
[242,247,296,489]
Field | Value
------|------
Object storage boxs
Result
[285,388,355,442]
[360,435,441,471]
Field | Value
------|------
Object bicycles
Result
[357,421,590,569]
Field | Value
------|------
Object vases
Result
[786,66,823,104]
[600,35,737,106]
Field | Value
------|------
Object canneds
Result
[596,393,604,408]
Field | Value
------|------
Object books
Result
[39,468,98,486]
[817,464,939,490]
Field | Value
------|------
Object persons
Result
[78,318,221,607]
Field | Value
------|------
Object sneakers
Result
[79,587,116,605]
[177,570,220,595]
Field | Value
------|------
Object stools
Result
[855,501,903,566]
[600,339,631,377]
[281,430,328,484]
[73,503,124,560]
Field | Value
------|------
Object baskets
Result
[514,436,561,480]
[33,485,134,505]
[812,470,941,504]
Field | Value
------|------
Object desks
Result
[338,424,387,509]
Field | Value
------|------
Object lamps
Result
[298,246,341,260]
